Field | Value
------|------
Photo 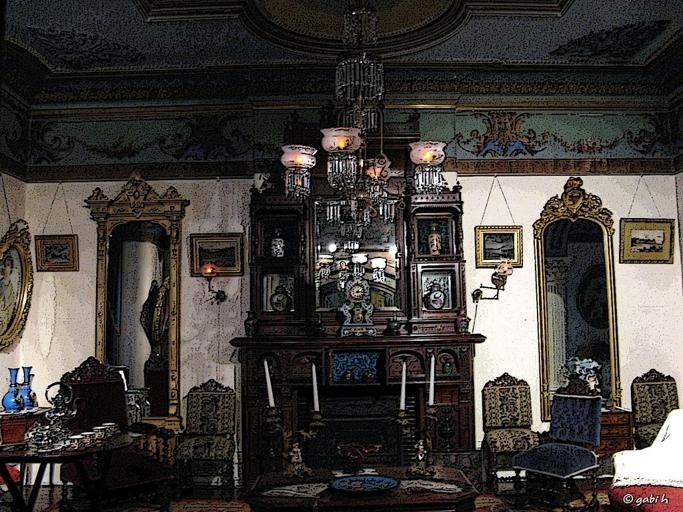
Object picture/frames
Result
[0,218,34,352]
[473,226,524,268]
[189,232,245,277]
[32,232,81,273]
[618,218,676,265]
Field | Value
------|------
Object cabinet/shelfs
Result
[0,406,51,443]
[227,326,488,452]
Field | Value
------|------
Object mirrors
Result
[82,168,191,438]
[531,176,624,423]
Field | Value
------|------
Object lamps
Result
[472,258,513,303]
[201,260,227,306]
[280,0,447,224]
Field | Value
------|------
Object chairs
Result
[173,379,237,503]
[480,367,682,512]
[59,357,177,512]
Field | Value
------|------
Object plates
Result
[330,473,399,496]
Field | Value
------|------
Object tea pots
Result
[45,381,80,433]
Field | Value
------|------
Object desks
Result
[246,465,480,509]
[0,432,134,512]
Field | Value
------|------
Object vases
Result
[143,339,169,417]
[1,366,38,413]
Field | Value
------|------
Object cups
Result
[68,435,85,450]
[93,425,110,441]
[80,430,99,447]
[103,422,118,436]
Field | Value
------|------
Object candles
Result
[312,362,319,411]
[264,358,275,407]
[428,354,436,404]
[399,361,406,411]
